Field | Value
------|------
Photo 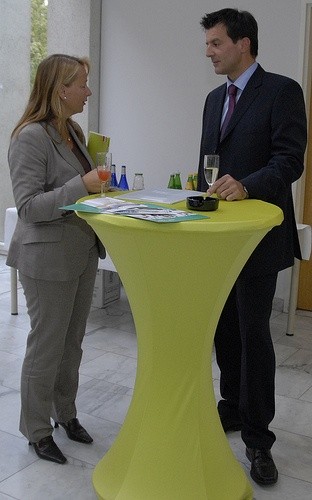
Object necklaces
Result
[60,125,71,142]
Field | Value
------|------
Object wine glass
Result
[204,155,220,197]
[95,153,112,198]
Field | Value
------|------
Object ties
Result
[220,85,237,143]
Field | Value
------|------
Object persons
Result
[4,54,106,464]
[196,9,309,483]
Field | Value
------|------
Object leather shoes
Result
[217,406,241,433]
[246,447,278,485]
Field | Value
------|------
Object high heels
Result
[54,418,93,444]
[28,435,67,464]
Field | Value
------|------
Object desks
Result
[75,190,284,500]
[4,207,312,336]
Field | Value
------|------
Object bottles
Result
[168,174,176,189]
[175,173,182,189]
[185,172,198,191]
[118,166,129,190]
[109,164,118,187]
[132,172,144,191]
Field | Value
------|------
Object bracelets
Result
[243,185,248,195]
